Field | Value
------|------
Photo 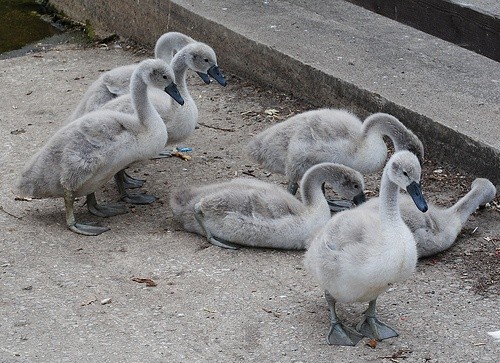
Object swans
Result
[241,107,426,212]
[355,177,499,257]
[68,31,198,163]
[101,41,228,206]
[169,161,368,250]
[12,56,186,237]
[302,151,427,348]
